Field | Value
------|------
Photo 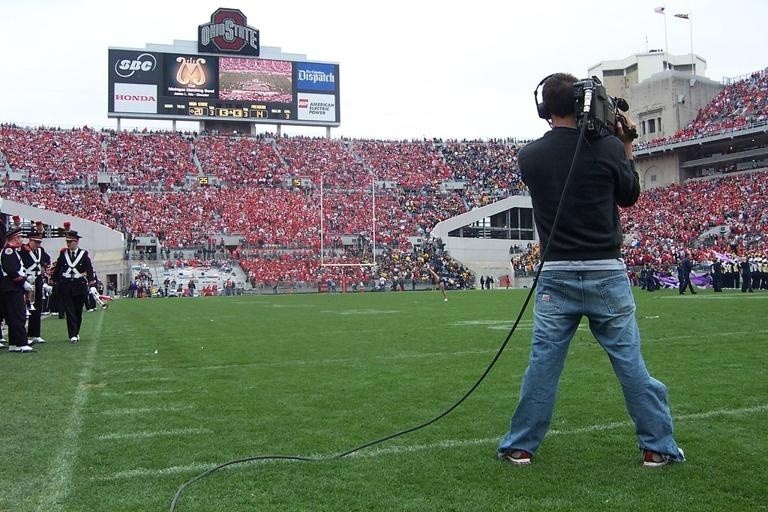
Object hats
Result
[27,233,44,242]
[65,231,82,242]
[723,257,767,264]
[5,226,23,239]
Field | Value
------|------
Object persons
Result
[631,67,767,153]
[497,73,689,468]
[2,228,103,352]
[1,126,766,298]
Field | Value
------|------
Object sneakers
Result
[0,337,45,352]
[71,334,79,343]
[40,311,59,316]
[87,307,97,312]
[644,448,684,466]
[508,449,533,467]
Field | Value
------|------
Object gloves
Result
[27,276,35,285]
[23,281,33,292]
[88,286,97,299]
[45,285,53,294]
